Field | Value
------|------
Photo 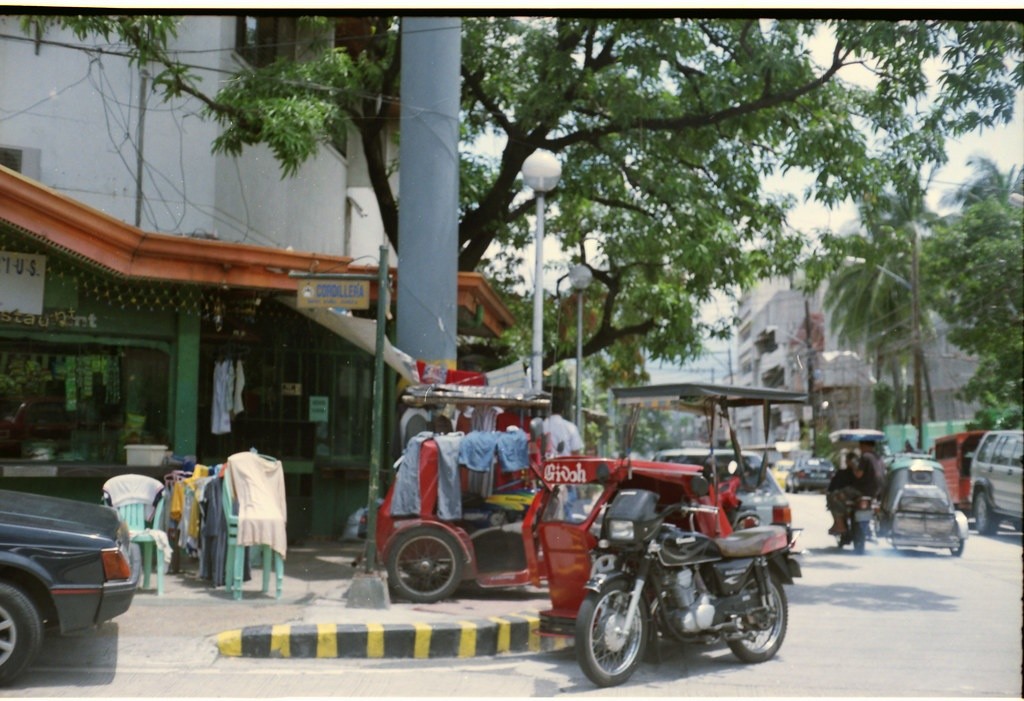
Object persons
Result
[542,396,584,457]
[826,440,886,535]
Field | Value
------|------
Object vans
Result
[962,427,1024,536]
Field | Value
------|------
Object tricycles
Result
[376,383,571,602]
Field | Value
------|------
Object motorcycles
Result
[576,473,808,688]
[836,496,877,557]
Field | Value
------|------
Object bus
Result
[922,431,993,515]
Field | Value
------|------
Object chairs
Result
[221,453,284,602]
[103,474,167,596]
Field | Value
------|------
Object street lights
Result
[570,264,593,437]
[521,146,564,398]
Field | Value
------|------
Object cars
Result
[0,485,147,690]
[787,456,836,495]
[650,447,794,545]
[741,443,788,490]
